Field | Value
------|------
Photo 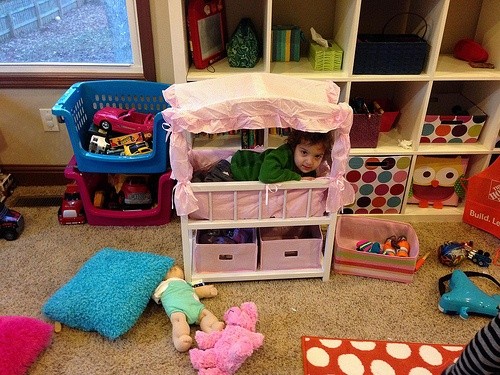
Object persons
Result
[190,129,334,183]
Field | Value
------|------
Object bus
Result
[87,135,110,154]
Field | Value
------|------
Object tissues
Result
[307,26,344,71]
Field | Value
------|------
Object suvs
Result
[0,201,27,243]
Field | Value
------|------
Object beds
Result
[162,78,354,285]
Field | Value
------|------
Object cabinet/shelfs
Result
[169,0,500,223]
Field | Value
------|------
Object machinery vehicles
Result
[108,173,154,210]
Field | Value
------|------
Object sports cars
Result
[93,106,154,135]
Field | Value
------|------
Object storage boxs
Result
[332,216,419,284]
[339,156,412,214]
[374,98,400,132]
[420,92,488,143]
[258,227,323,270]
[353,33,431,75]
[193,229,258,272]
[349,99,383,148]
[407,156,469,207]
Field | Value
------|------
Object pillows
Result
[0,315,54,375]
[41,247,174,340]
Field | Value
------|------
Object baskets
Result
[354,12,429,75]
[51,80,173,174]
[64,154,175,227]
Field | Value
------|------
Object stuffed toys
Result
[151,264,225,352]
[189,301,265,375]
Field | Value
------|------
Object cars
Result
[57,182,87,226]
[123,141,153,156]
